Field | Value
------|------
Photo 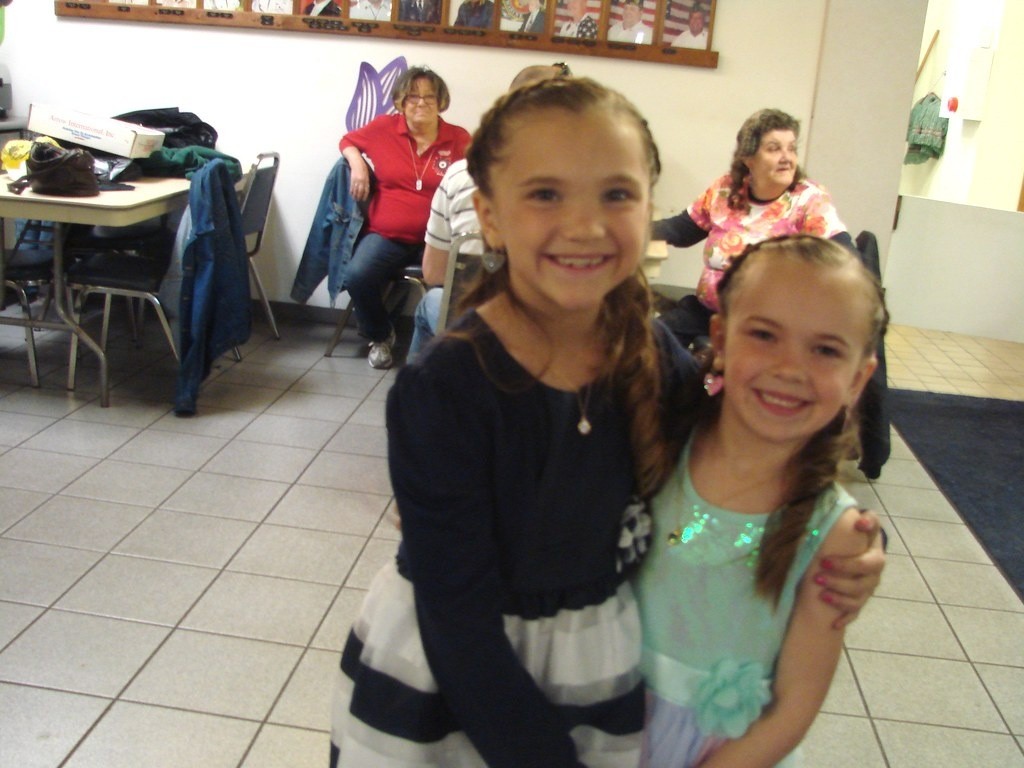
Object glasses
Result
[405,94,437,105]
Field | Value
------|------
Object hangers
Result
[918,92,938,106]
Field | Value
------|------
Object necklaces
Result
[667,418,811,545]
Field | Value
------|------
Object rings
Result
[365,184,370,188]
[354,183,359,187]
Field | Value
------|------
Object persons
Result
[160,1,196,11]
[452,0,495,30]
[643,107,851,392]
[301,1,343,19]
[325,77,891,768]
[605,0,657,46]
[403,58,594,386]
[515,0,547,35]
[557,1,600,43]
[400,0,440,24]
[252,0,293,16]
[349,0,392,22]
[203,0,240,12]
[337,64,476,371]
[620,231,890,768]
[668,5,714,51]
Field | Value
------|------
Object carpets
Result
[883,387,1023,608]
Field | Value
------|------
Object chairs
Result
[325,157,428,357]
[64,159,230,403]
[2,248,75,388]
[436,230,487,336]
[233,151,281,361]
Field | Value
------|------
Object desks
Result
[643,196,669,281]
[0,165,253,416]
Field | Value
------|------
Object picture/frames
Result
[54,0,719,69]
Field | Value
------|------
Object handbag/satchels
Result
[7,141,99,198]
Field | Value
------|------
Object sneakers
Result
[368,320,396,371]
